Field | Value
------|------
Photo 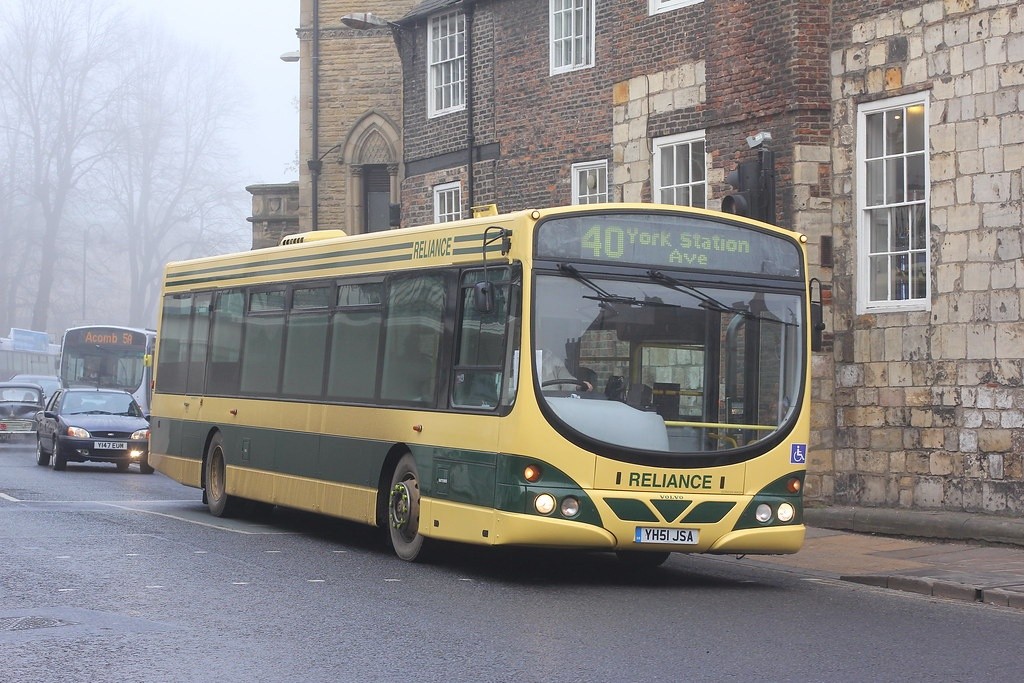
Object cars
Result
[35,388,154,474]
[8,374,64,406]
[0,382,46,442]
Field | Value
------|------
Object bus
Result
[147,200,825,572]
[59,325,157,423]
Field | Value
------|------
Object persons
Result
[496,329,593,405]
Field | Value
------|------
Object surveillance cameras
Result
[747,133,772,148]
